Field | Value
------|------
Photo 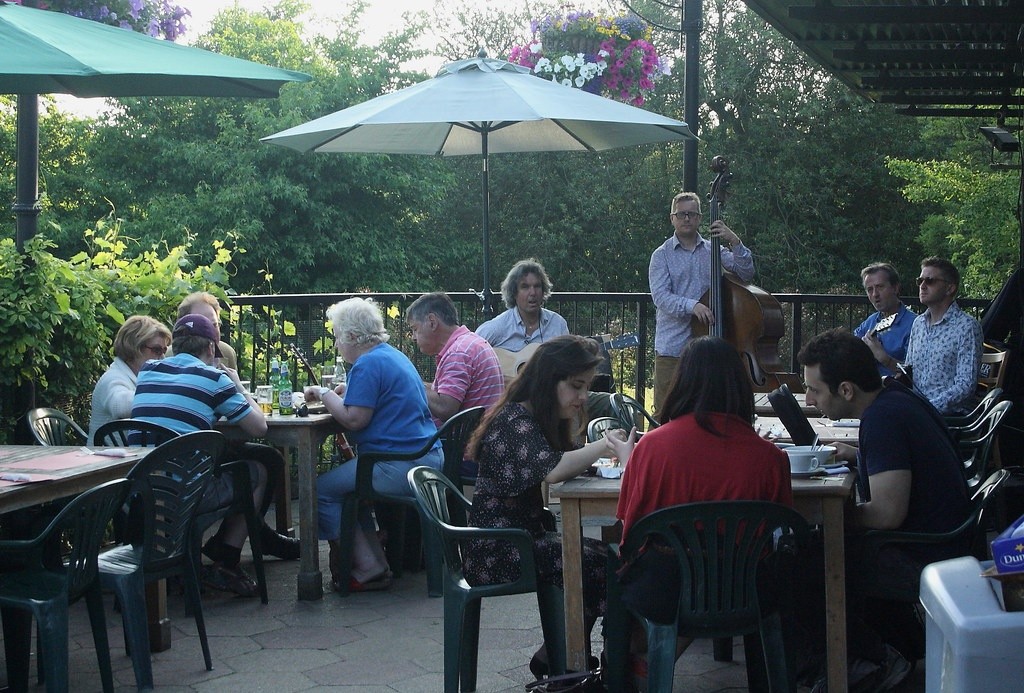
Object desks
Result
[550,443,858,693]
[215,411,351,601]
[752,417,861,449]
[754,391,825,418]
[0,444,172,655]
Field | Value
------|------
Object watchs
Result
[319,387,331,401]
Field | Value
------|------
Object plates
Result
[293,405,326,414]
[790,467,825,476]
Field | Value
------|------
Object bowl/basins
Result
[782,446,837,465]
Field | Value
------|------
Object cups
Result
[291,391,304,402]
[788,455,820,473]
[234,381,251,401]
[321,365,335,390]
[303,386,322,394]
[214,358,228,369]
[257,385,273,419]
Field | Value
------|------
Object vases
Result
[543,34,600,54]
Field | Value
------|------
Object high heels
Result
[530,655,598,688]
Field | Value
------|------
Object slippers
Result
[332,575,391,590]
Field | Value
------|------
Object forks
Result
[80,446,138,456]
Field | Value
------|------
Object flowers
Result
[0,0,192,42]
[508,11,676,106]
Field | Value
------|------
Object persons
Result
[403,292,505,477]
[302,297,444,591]
[787,328,971,693]
[474,259,570,351]
[854,262,919,377]
[896,257,985,414]
[649,192,754,432]
[601,334,792,693]
[87,291,301,595]
[463,335,626,680]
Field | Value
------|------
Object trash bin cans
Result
[919,555,1024,693]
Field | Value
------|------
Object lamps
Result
[979,127,1024,170]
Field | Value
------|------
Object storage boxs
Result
[980,514,1024,612]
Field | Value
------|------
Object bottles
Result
[268,358,281,409]
[279,361,292,415]
[332,356,346,400]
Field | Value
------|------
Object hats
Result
[172,313,224,358]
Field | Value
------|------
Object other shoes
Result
[874,643,911,693]
[810,658,880,693]
[261,526,301,559]
[200,562,259,596]
[202,535,218,561]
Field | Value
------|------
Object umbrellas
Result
[0,0,314,101]
[259,52,702,322]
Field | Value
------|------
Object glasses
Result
[916,277,946,286]
[146,345,167,355]
[671,211,702,219]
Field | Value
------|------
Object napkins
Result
[825,466,850,474]
[833,419,860,426]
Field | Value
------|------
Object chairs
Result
[0,408,268,693]
[407,344,1009,693]
[339,406,486,597]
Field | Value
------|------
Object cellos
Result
[691,154,805,394]
